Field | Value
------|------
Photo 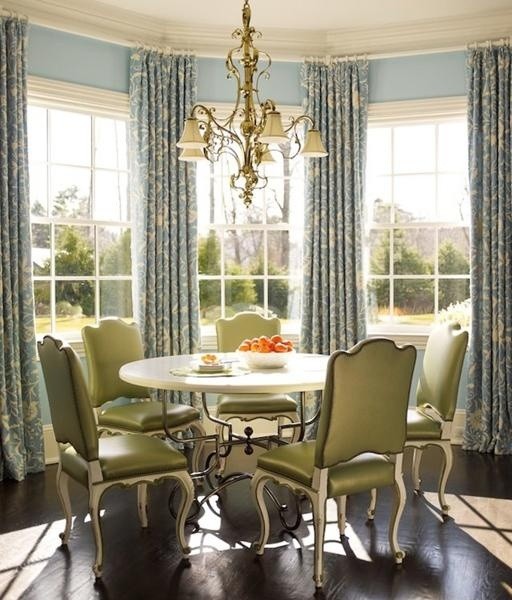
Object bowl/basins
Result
[236,349,296,369]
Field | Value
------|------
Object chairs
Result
[252,338,417,587]
[35,336,195,580]
[214,310,300,473]
[80,317,206,528]
[363,318,468,517]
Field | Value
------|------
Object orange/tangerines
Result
[239,335,293,352]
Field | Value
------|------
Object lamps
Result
[175,0,330,210]
